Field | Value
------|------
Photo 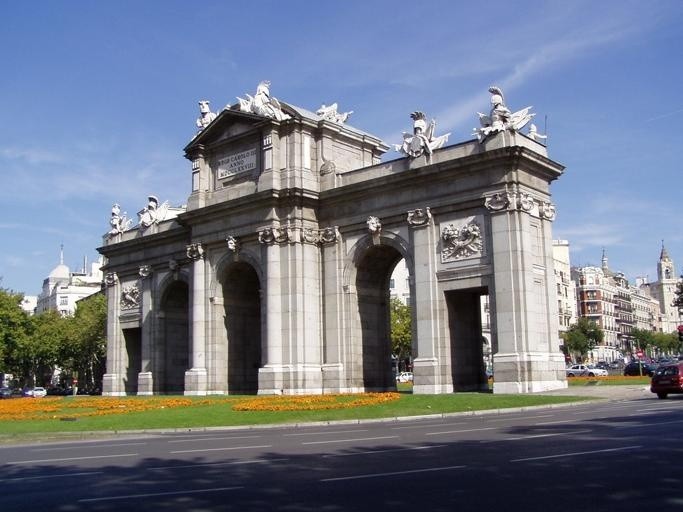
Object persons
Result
[71,374,79,395]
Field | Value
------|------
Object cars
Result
[0,386,102,399]
[394,371,413,383]
[484,368,493,379]
[565,357,683,400]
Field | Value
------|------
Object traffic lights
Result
[636,351,642,357]
[677,325,683,342]
[72,379,77,383]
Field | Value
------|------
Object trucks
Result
[0,373,13,387]
